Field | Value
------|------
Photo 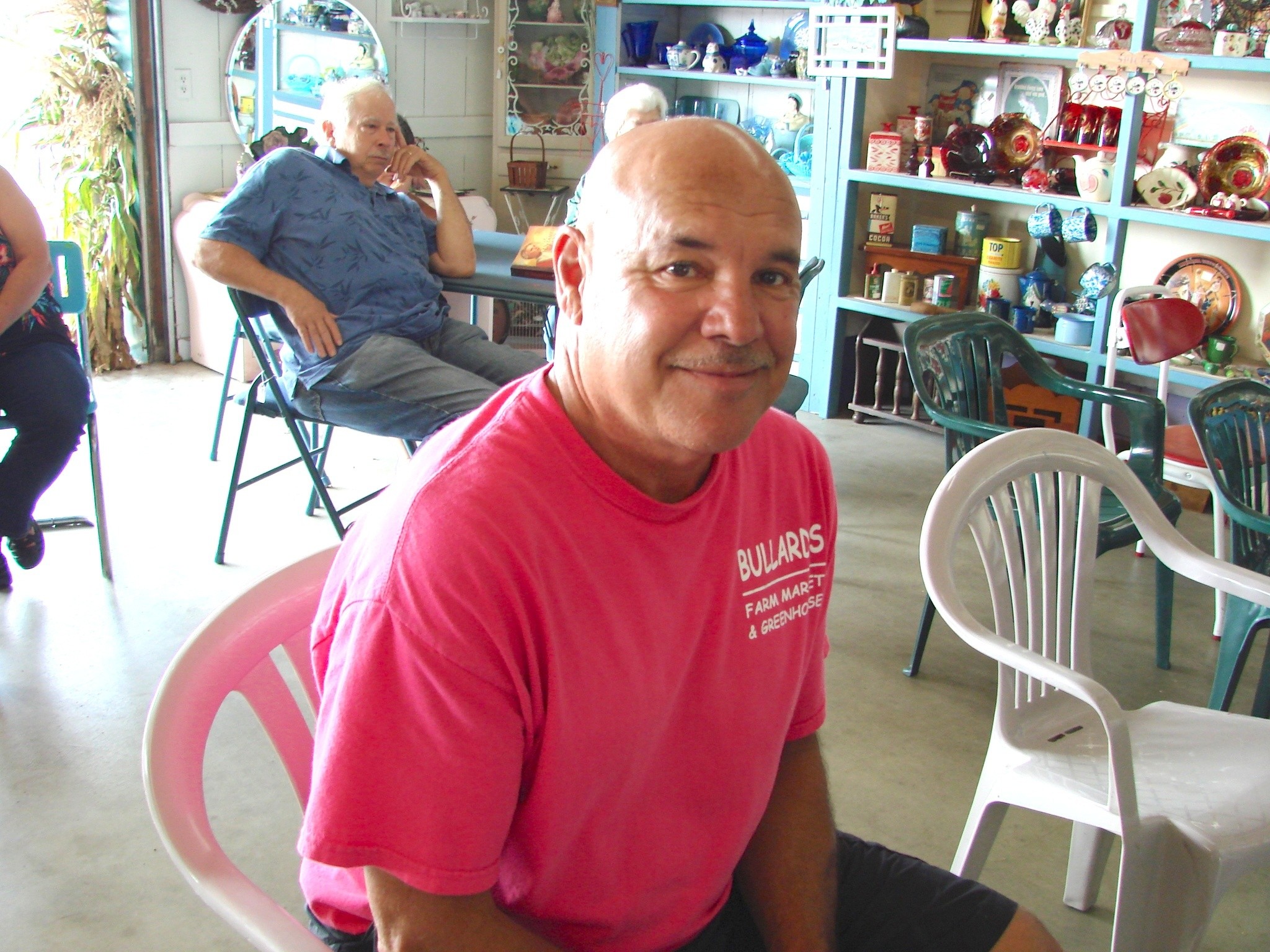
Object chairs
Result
[143,544,343,952]
[903,285,1270,952]
[0,242,114,580]
[210,284,418,565]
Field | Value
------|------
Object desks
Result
[500,184,569,237]
[440,229,560,326]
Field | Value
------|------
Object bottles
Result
[866,105,932,175]
[975,266,1025,313]
[862,263,920,307]
[733,18,768,64]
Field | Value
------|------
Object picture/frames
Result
[966,0,1092,48]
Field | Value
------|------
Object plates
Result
[686,10,809,54]
[1135,167,1199,209]
[1149,253,1241,345]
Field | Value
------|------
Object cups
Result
[1214,29,1256,57]
[986,297,1010,321]
[1078,262,1118,299]
[1056,102,1121,146]
[1207,334,1239,363]
[1027,202,1098,244]
[1013,306,1037,334]
[654,43,675,65]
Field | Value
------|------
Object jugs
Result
[666,40,701,71]
[1149,142,1206,179]
[1018,266,1066,328]
[621,20,658,67]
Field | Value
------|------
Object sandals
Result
[9,517,45,569]
[0,552,12,586]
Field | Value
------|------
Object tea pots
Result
[1072,150,1116,201]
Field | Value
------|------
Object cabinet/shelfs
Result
[817,0,1270,514]
[488,0,849,415]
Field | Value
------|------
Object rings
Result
[407,150,412,156]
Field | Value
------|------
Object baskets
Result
[507,130,548,189]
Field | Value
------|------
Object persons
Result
[565,83,669,226]
[193,78,551,441]
[296,117,1060,951]
[0,164,90,590]
[377,113,436,218]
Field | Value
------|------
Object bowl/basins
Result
[1199,135,1270,200]
[1054,313,1094,345]
[1153,4,1213,53]
